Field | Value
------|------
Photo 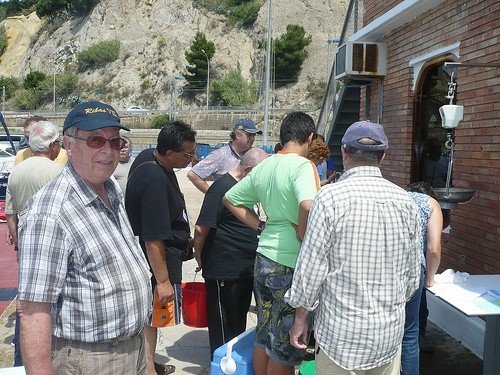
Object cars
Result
[125,106,152,115]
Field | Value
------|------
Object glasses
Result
[67,134,127,149]
[184,150,199,157]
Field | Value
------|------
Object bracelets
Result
[256,220,266,236]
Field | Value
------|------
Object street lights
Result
[201,49,210,111]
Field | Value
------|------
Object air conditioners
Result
[333,40,388,88]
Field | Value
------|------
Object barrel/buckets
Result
[181,269,210,329]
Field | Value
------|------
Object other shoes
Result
[153,361,176,375]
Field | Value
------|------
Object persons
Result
[307,133,342,188]
[16,100,156,375]
[219,111,324,374]
[284,118,422,375]
[193,145,270,363]
[4,117,70,347]
[125,119,199,375]
[418,138,451,188]
[185,116,265,194]
[3,120,68,368]
[398,181,443,375]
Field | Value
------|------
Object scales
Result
[435,62,500,202]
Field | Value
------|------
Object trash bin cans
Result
[194,142,208,166]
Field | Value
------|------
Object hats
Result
[341,120,390,151]
[233,117,264,136]
[62,100,130,135]
